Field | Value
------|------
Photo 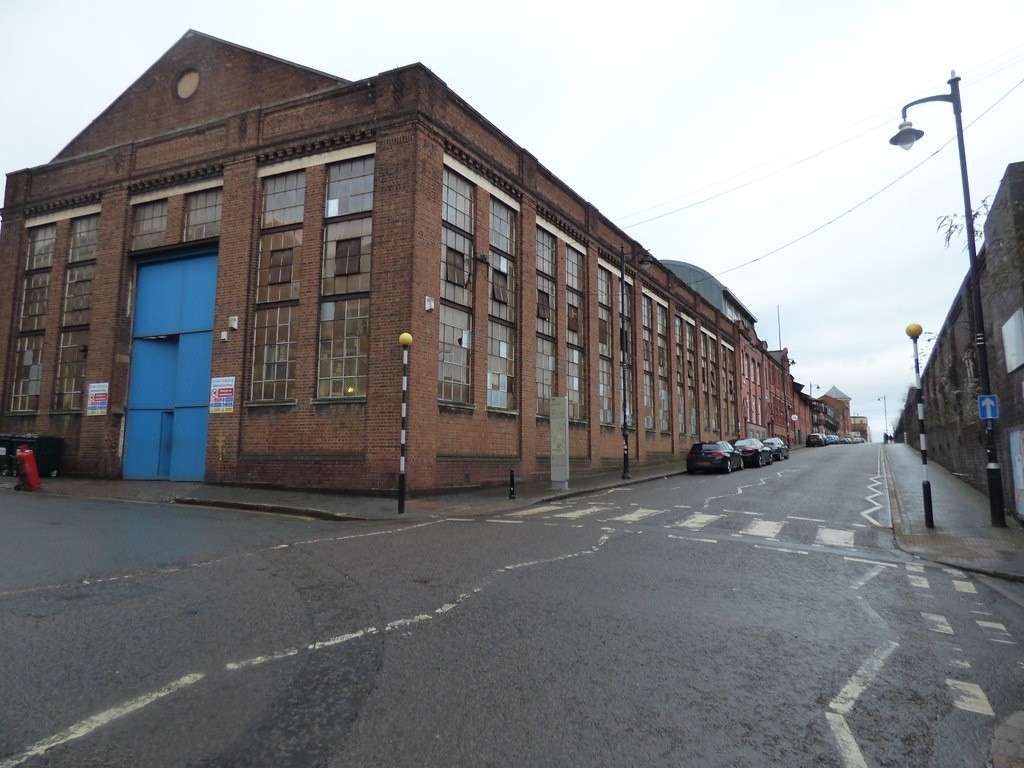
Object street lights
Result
[887,71,1007,527]
[878,396,888,444]
[781,357,797,448]
[810,382,821,432]
[904,323,934,528]
[617,242,654,480]
[397,333,413,514]
[853,412,859,431]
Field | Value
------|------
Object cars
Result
[836,435,839,440]
[837,438,848,444]
[728,438,773,467]
[686,440,744,474]
[826,435,835,444]
[853,438,866,443]
[760,437,790,461]
[820,434,829,445]
[832,436,837,443]
[847,437,852,444]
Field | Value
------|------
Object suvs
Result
[806,434,825,447]
